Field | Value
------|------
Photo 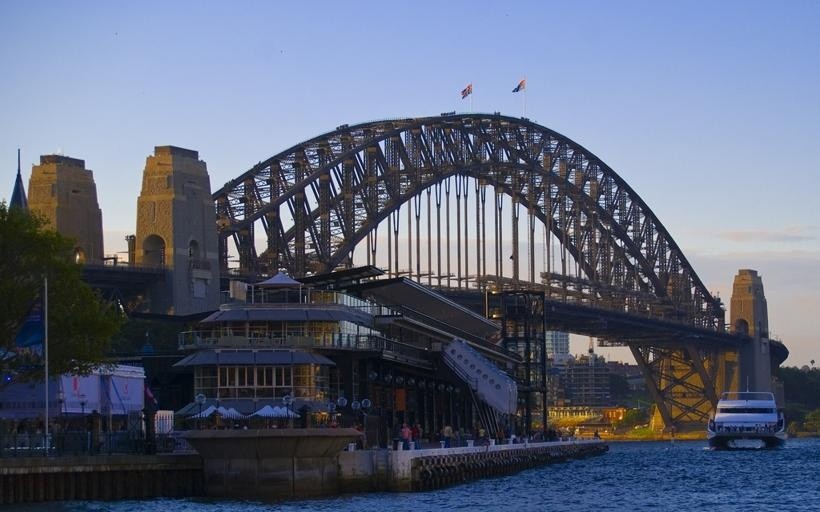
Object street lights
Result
[362,399,371,450]
[194,394,207,430]
[79,394,88,432]
[57,391,66,433]
[337,398,347,428]
[283,395,292,429]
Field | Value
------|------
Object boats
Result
[707,376,789,450]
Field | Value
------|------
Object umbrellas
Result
[186,404,302,418]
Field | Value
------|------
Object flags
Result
[16,289,46,348]
[512,79,525,93]
[461,83,471,100]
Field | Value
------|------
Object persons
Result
[183,414,293,431]
[399,415,602,450]
[0,414,128,455]
